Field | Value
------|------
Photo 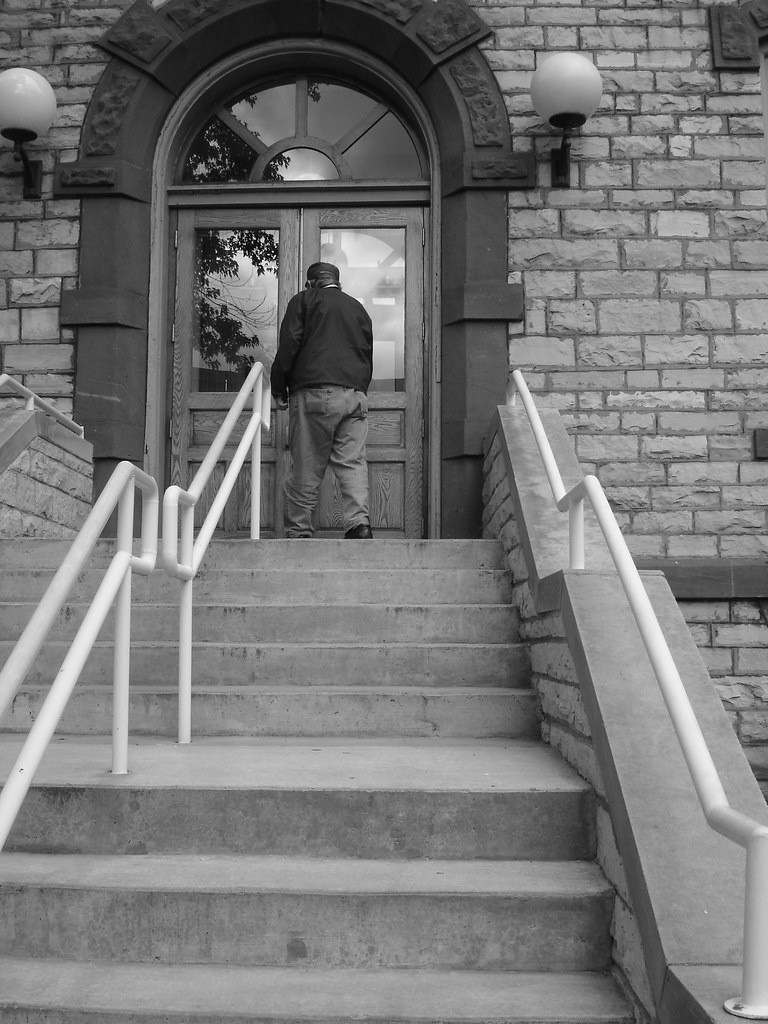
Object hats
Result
[304,262,339,288]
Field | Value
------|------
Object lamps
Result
[0,67,57,200]
[530,53,603,189]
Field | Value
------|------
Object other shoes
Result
[344,523,372,539]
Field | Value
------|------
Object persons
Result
[270,262,374,539]
